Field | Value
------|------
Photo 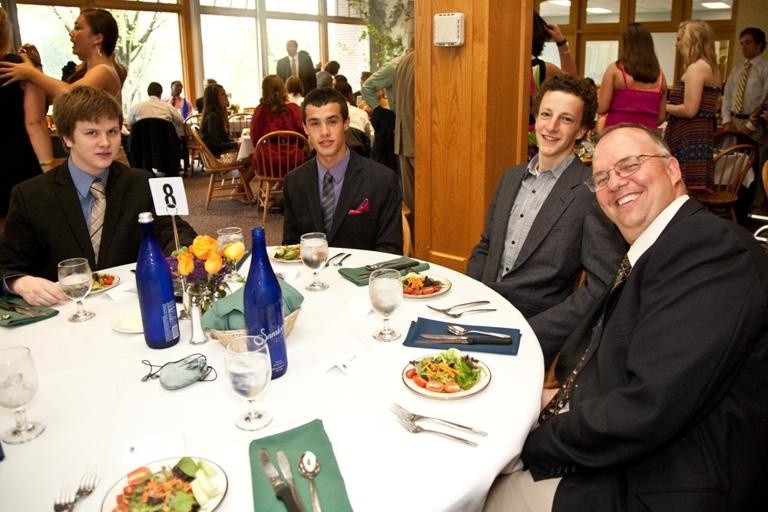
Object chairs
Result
[112,102,377,227]
[681,141,751,223]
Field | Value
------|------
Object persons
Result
[595,23,670,135]
[282,85,406,259]
[466,78,626,372]
[530,10,578,155]
[2,5,259,214]
[485,126,768,511]
[720,27,768,213]
[665,21,719,200]
[250,37,417,213]
[0,85,198,309]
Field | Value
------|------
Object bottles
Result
[242,226,287,380]
[189,296,209,345]
[132,210,181,352]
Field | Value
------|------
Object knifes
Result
[356,260,418,283]
[258,449,301,512]
[414,332,512,346]
[0,300,49,318]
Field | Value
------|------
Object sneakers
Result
[230,189,258,204]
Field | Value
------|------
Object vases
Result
[180,278,224,319]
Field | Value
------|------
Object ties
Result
[320,172,335,234]
[292,59,295,76]
[89,182,107,263]
[538,254,633,423]
[171,98,176,105]
[734,63,752,114]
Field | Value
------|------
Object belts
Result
[731,111,749,119]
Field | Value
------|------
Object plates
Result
[108,301,180,334]
[85,272,121,296]
[100,455,230,512]
[402,274,451,299]
[268,244,301,263]
[400,352,492,401]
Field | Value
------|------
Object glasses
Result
[586,154,667,193]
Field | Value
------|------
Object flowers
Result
[163,233,246,288]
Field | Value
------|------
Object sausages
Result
[116,467,155,510]
[406,367,462,393]
[403,286,439,295]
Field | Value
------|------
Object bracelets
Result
[556,39,569,51]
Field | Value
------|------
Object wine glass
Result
[56,257,96,324]
[224,336,274,433]
[216,226,246,284]
[0,346,48,446]
[299,232,329,292]
[368,268,403,342]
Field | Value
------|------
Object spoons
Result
[445,325,512,337]
[297,450,323,512]
[364,258,411,271]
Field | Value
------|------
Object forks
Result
[396,414,479,449]
[440,308,497,319]
[389,401,489,439]
[425,300,490,314]
[52,482,74,512]
[69,472,101,512]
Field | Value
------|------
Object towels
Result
[0,298,59,328]
[401,312,523,358]
[336,256,431,287]
[247,418,352,511]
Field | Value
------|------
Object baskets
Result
[210,305,300,354]
